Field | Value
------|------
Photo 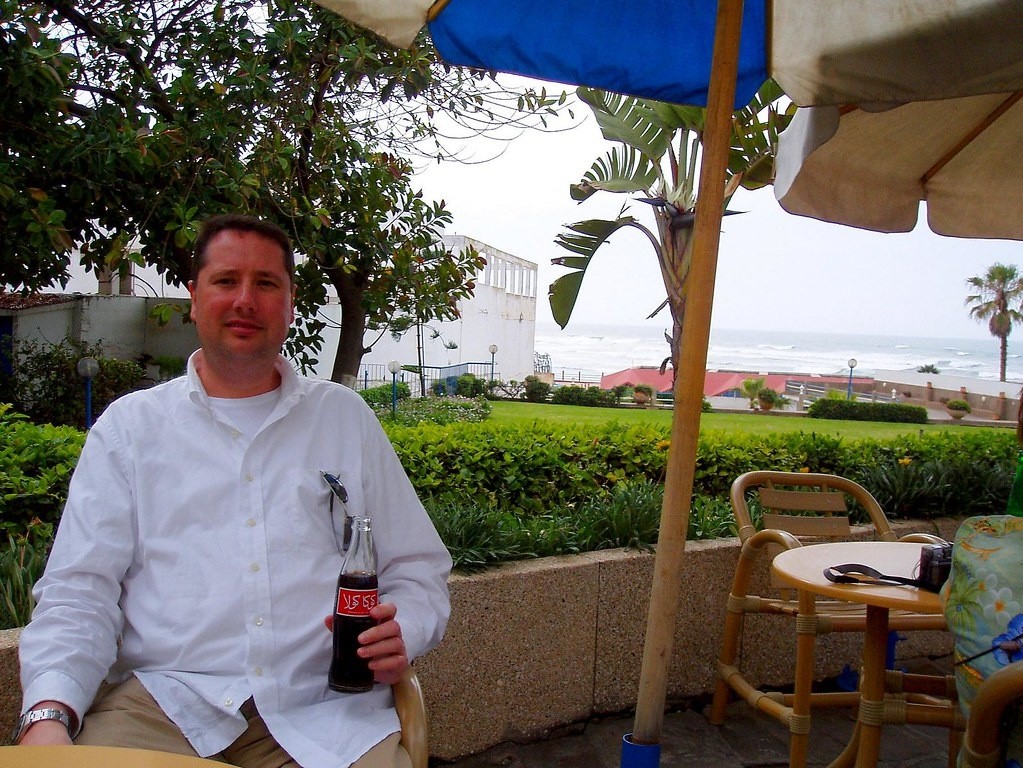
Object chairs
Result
[709,470,949,768]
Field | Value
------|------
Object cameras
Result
[919,541,954,589]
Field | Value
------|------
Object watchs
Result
[11,708,71,746]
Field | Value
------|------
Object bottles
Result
[326,517,381,695]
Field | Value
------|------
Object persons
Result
[14,214,455,768]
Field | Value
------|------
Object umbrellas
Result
[320,0,1023,768]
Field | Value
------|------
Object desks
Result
[770,542,969,768]
